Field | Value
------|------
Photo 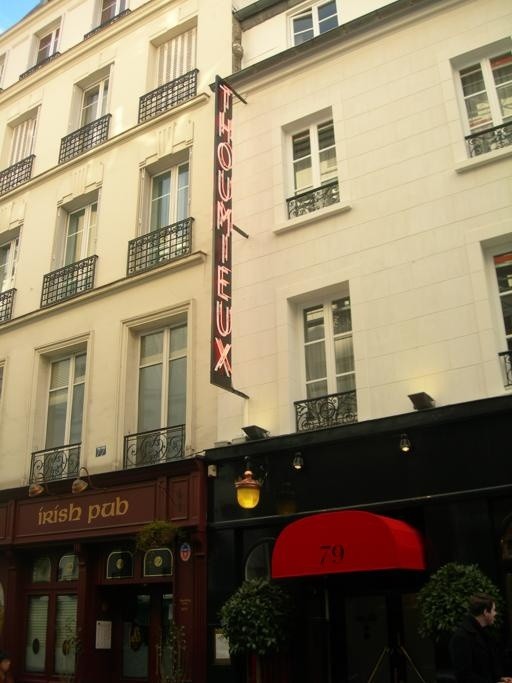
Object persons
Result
[445,592,511,682]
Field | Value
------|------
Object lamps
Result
[30,467,105,495]
[293,449,304,470]
[235,457,261,509]
[400,432,410,452]
